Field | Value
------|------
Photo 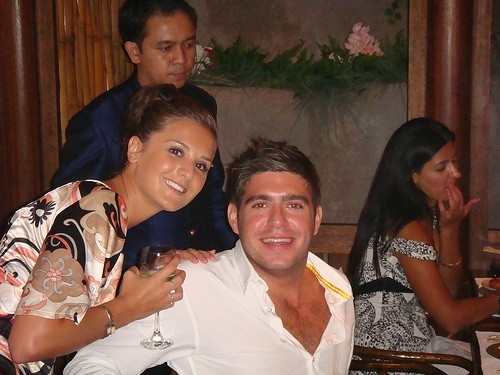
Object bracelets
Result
[97,305,115,339]
[439,257,462,268]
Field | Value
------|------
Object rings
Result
[171,302,173,307]
[170,293,174,301]
[170,280,177,290]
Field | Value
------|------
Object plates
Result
[481,279,497,291]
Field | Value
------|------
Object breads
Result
[489,277,500,289]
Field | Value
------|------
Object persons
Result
[51,0,238,298]
[62,136,355,375]
[345,117,500,375]
[0,82,218,375]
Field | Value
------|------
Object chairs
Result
[335,263,474,375]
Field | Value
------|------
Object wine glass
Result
[139,243,175,350]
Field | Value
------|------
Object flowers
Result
[190,0,408,148]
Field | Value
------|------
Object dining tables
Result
[467,271,500,375]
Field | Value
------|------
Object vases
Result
[196,82,407,224]
